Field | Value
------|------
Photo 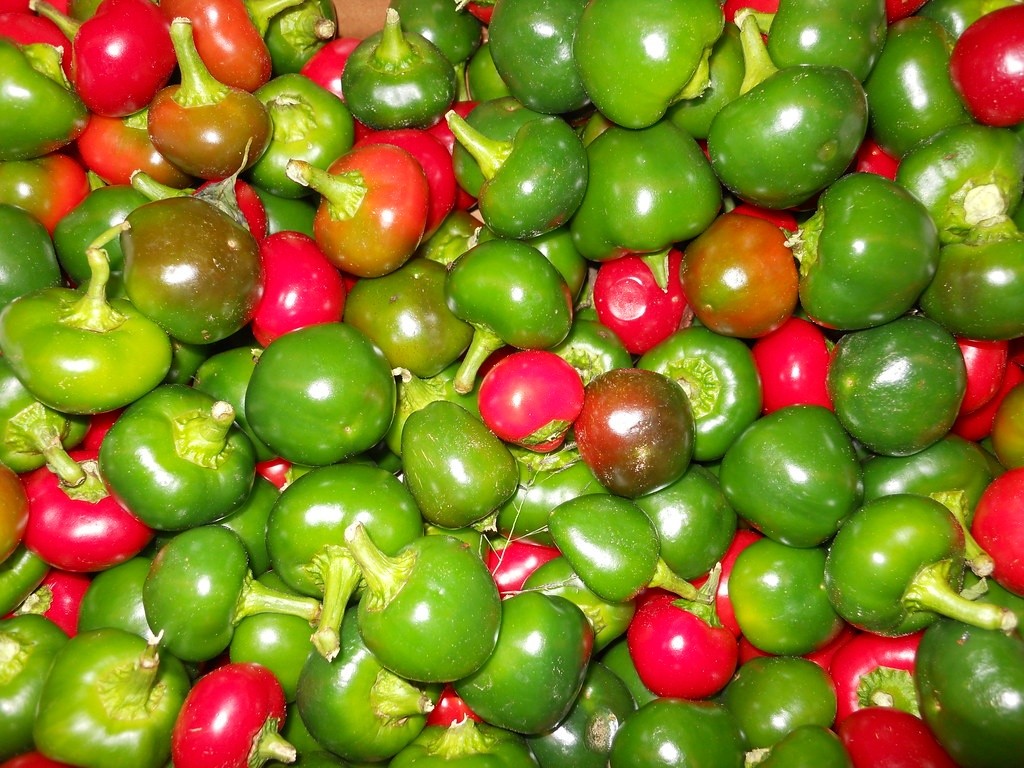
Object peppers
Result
[0,0,1024,768]
